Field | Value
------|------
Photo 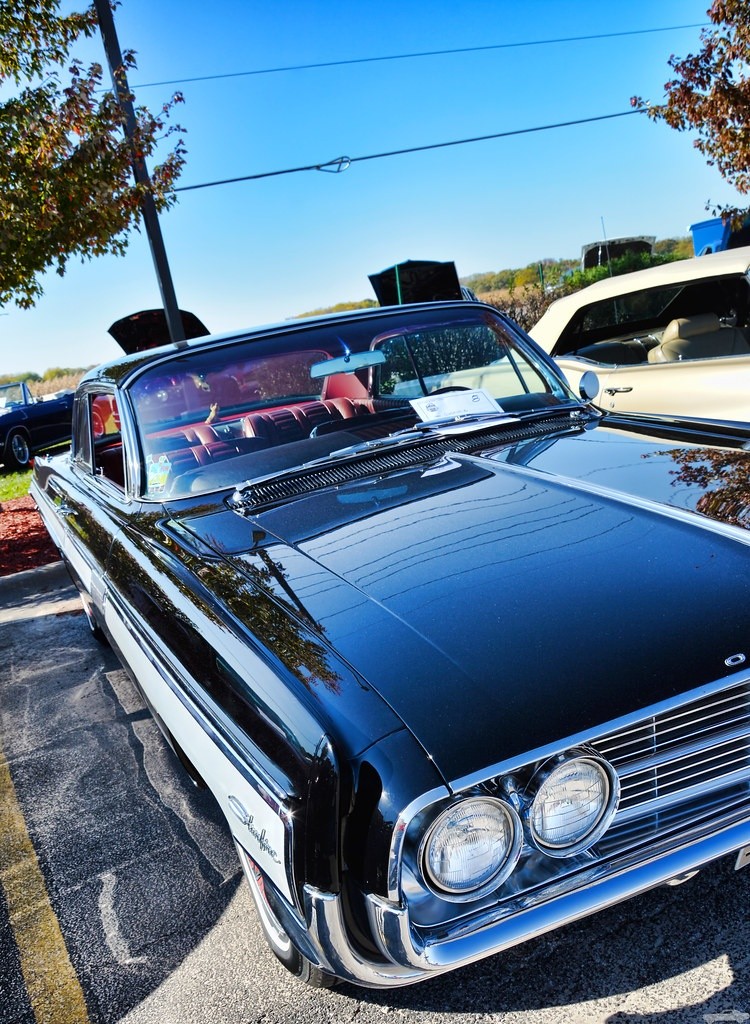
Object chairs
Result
[138,435,269,488]
[100,423,227,495]
[243,396,360,443]
[647,311,750,366]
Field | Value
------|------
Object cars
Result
[389,240,750,430]
[107,306,210,360]
[370,258,478,316]
[549,233,664,301]
[26,300,747,994]
[690,218,746,258]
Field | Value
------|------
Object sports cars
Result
[0,382,77,466]
